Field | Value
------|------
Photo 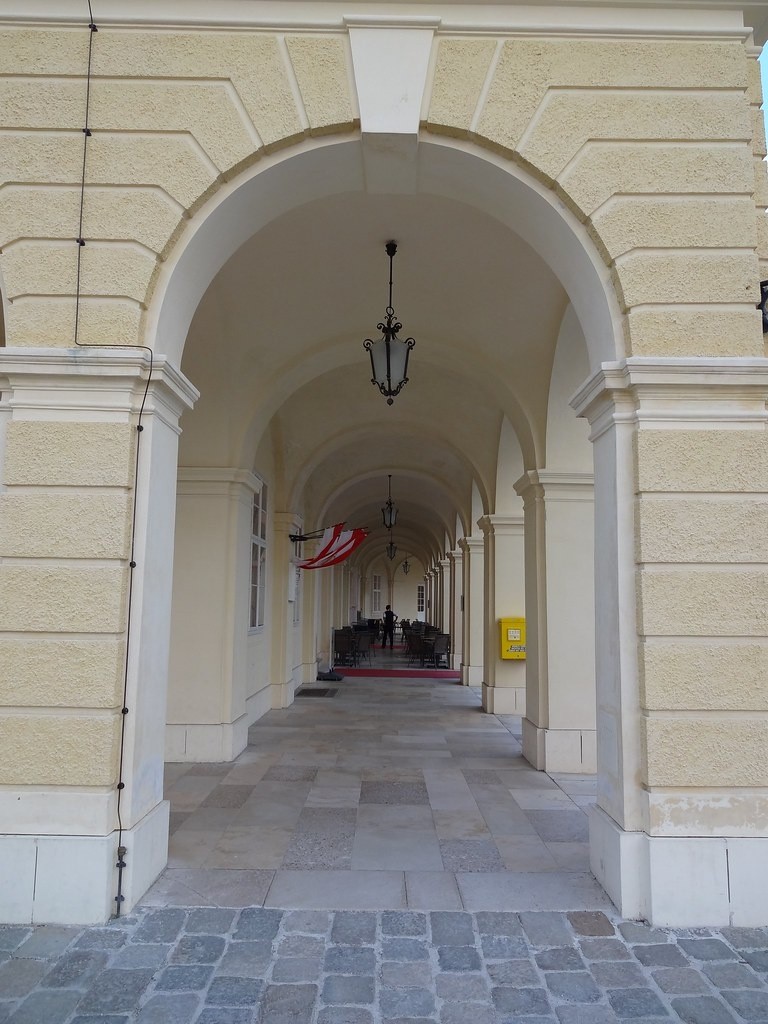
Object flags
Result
[287,522,371,569]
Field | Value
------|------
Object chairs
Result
[334,617,451,669]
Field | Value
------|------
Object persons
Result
[380,604,398,649]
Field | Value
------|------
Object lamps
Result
[402,550,411,575]
[386,531,398,562]
[362,238,416,407]
[381,474,399,530]
